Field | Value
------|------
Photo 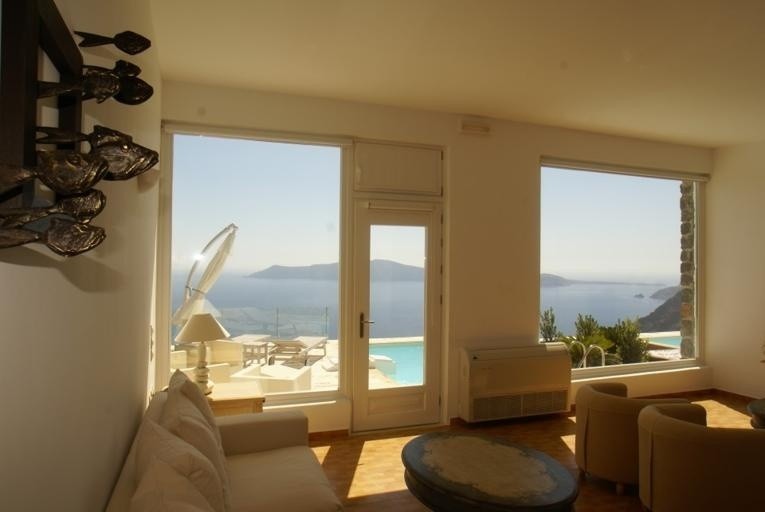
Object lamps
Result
[174,312,231,396]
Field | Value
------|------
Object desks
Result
[242,341,267,368]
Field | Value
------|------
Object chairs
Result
[575,378,765,512]
[268,334,327,367]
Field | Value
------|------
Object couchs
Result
[104,379,345,512]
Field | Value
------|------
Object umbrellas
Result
[172,222,238,325]
